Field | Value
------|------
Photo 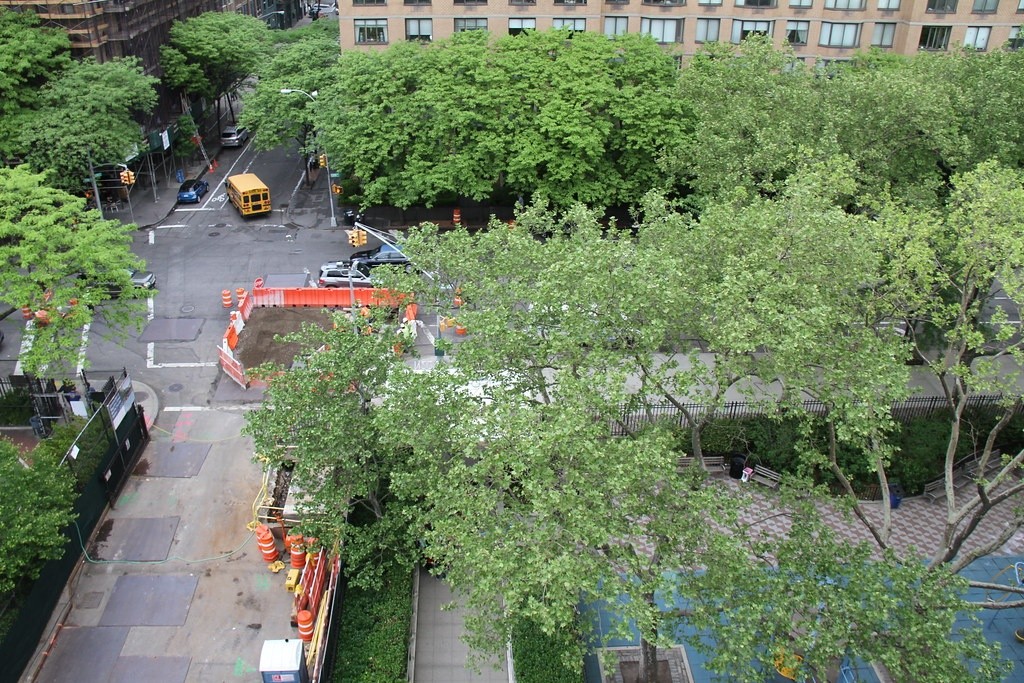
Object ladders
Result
[182,98,212,168]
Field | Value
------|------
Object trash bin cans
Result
[356,212,365,223]
[344,210,353,224]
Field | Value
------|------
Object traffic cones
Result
[213,159,219,167]
[22,305,32,318]
[209,164,214,172]
[454,288,464,305]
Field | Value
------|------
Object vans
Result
[522,301,645,349]
[220,127,248,148]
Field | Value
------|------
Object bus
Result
[224,173,272,219]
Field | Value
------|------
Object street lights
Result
[336,261,360,335]
[281,89,339,227]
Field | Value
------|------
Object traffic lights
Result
[351,233,358,247]
[123,172,129,184]
[129,172,135,183]
[319,154,326,166]
[120,172,124,184]
[359,230,367,245]
[348,231,353,244]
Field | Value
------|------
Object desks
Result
[741,466,752,482]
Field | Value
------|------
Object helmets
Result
[402,318,408,324]
[400,323,405,328]
[359,303,362,308]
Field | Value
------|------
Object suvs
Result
[74,260,157,299]
[349,243,427,275]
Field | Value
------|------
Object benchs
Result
[923,448,1003,502]
[750,463,781,489]
[674,455,725,477]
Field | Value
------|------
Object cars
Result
[318,261,388,288]
[314,4,339,15]
[177,179,210,204]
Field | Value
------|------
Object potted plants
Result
[1015,628,1024,641]
